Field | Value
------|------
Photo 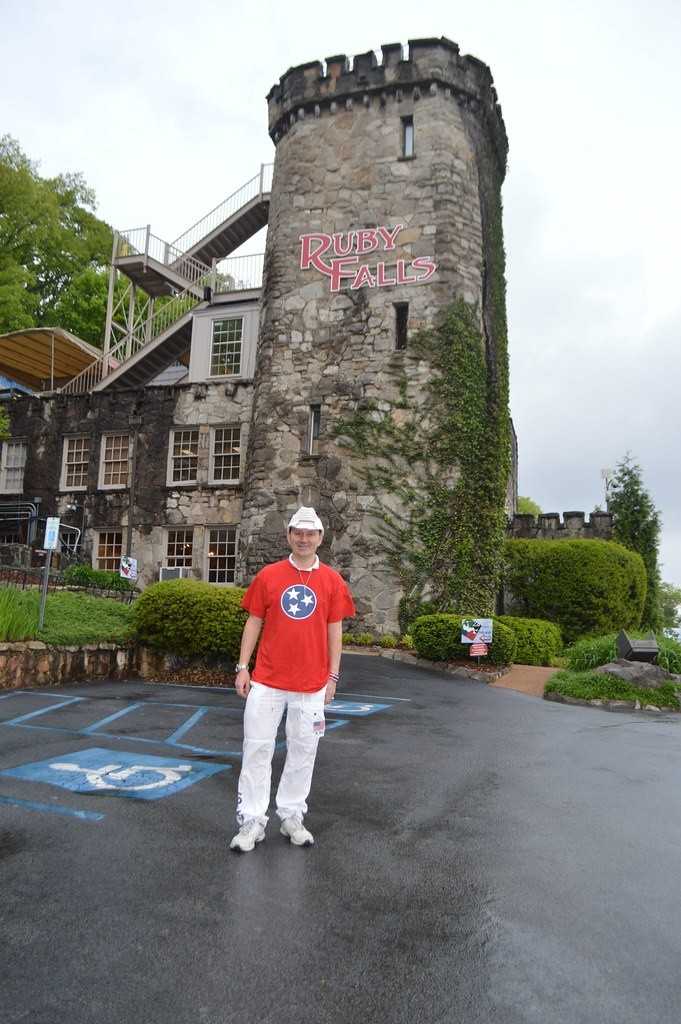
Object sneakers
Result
[280,817,314,848]
[229,819,265,853]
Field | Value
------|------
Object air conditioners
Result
[159,566,190,585]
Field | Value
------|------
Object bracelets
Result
[329,673,339,683]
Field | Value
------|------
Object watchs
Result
[235,664,250,672]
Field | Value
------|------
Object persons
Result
[229,505,355,852]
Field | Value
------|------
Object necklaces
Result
[298,569,312,590]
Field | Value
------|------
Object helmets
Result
[287,507,324,536]
